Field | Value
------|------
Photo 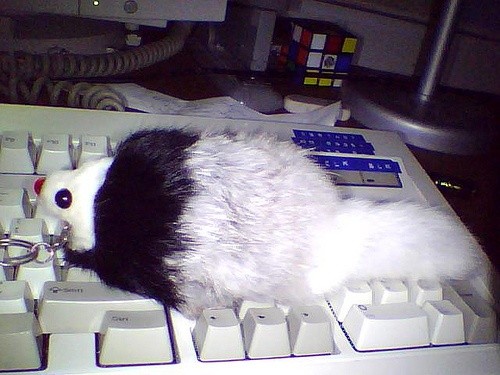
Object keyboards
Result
[0,102,499,374]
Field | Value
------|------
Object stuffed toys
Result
[34,127,489,321]
